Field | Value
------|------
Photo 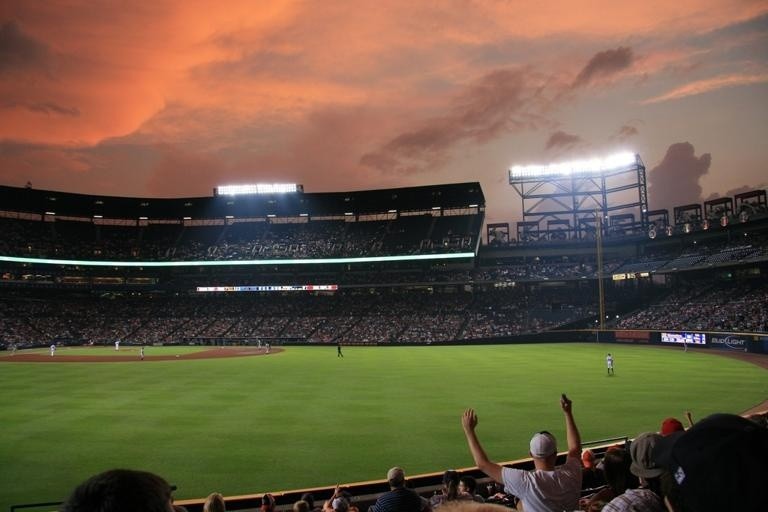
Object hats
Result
[332,497,348,509]
[262,494,275,505]
[630,413,768,511]
[581,448,596,461]
[529,431,557,458]
[387,467,404,483]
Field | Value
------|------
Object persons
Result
[607,354,615,374]
[651,412,768,512]
[763,412,768,423]
[60,469,176,512]
[749,415,768,428]
